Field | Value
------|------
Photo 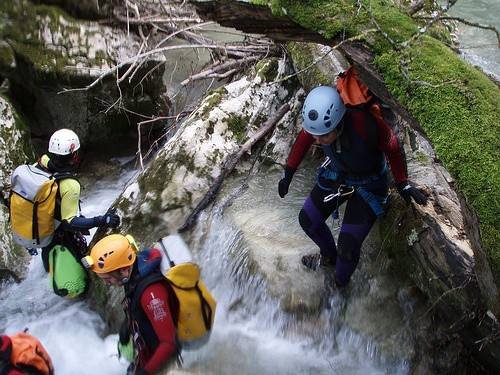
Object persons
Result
[80,234,183,375]
[0,335,26,375]
[31,128,121,273]
[277,86,428,288]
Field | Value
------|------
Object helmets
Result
[48,129,80,155]
[301,86,346,135]
[90,235,136,274]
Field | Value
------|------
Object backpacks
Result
[132,235,216,356]
[335,66,400,141]
[6,331,55,375]
[7,159,79,253]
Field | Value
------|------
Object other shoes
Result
[302,254,335,269]
[331,286,348,311]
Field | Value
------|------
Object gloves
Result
[95,208,121,228]
[278,171,294,199]
[397,181,428,207]
